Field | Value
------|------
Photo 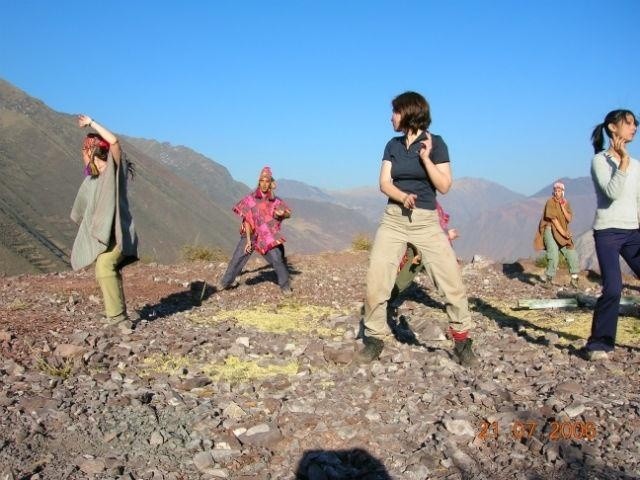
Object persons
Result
[359,93,477,368]
[534,181,581,289]
[69,114,137,326]
[216,167,292,297]
[584,110,639,362]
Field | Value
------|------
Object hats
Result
[554,183,564,189]
[259,166,273,184]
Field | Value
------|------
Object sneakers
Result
[545,279,553,289]
[571,277,579,288]
[452,338,479,370]
[357,336,384,363]
[282,287,293,297]
[110,313,133,329]
[586,349,607,361]
[217,282,224,291]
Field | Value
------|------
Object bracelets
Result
[89,120,93,127]
